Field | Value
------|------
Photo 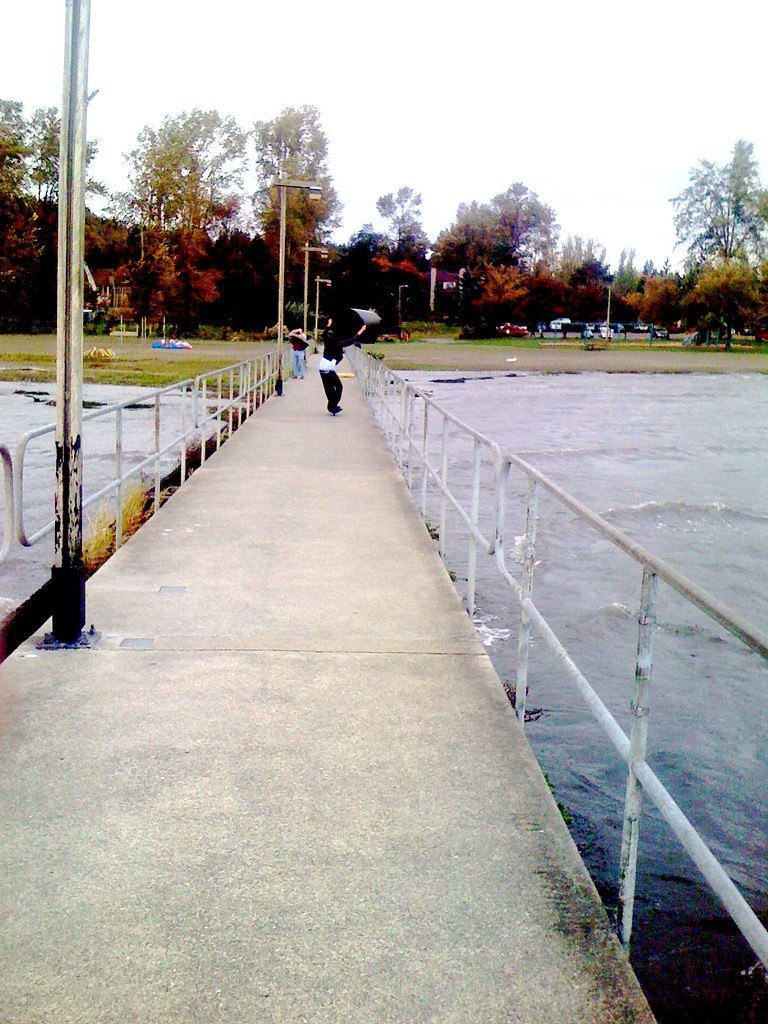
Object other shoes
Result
[301,375,304,379]
[328,406,343,417]
[292,376,297,379]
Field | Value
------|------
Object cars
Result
[496,324,529,337]
[653,326,669,340]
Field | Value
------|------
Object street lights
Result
[398,285,409,326]
[299,241,329,363]
[275,180,326,399]
[313,275,332,357]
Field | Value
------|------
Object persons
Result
[288,329,310,380]
[318,318,367,416]
